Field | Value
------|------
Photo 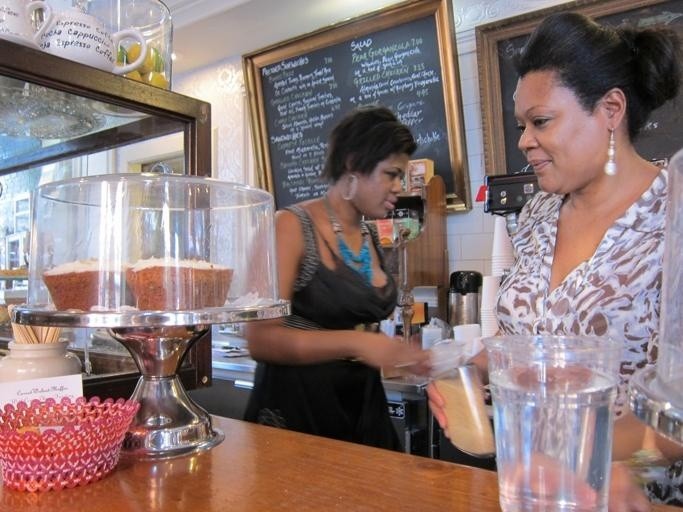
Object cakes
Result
[42,256,234,314]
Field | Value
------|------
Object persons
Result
[244,103,429,449]
[426,10,683,512]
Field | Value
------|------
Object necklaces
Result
[323,193,373,289]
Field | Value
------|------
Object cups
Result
[474,331,621,512]
[481,215,514,346]
[429,340,492,459]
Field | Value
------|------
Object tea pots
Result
[44,4,147,74]
[0,1,53,41]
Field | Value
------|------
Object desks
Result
[1,392,680,510]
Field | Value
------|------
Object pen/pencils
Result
[214,346,239,350]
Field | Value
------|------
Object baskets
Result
[2,395,141,493]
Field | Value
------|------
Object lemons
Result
[117,43,168,90]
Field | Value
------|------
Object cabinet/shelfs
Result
[0,39,215,408]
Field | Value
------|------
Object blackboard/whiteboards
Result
[242,1,472,215]
[474,1,683,174]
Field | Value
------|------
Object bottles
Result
[382,320,394,337]
[0,341,83,413]
[446,270,482,327]
[421,316,441,352]
